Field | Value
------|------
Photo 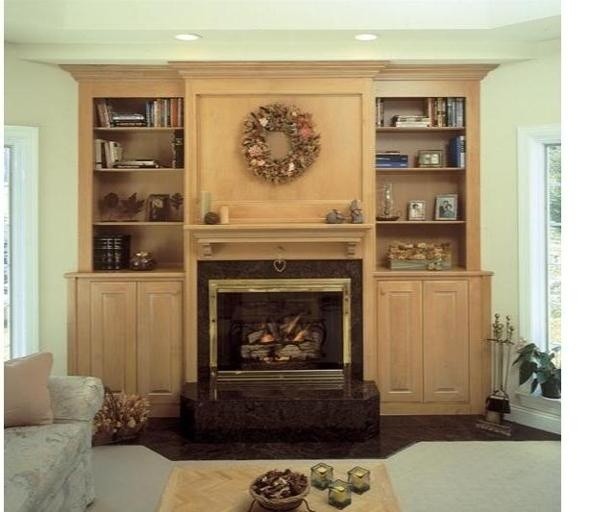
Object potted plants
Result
[512,341,561,399]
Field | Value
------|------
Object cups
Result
[197,190,210,222]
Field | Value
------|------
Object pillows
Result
[3,350,59,427]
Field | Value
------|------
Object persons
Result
[413,203,422,216]
[439,200,454,217]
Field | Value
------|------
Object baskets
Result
[249,470,310,510]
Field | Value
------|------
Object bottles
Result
[381,180,394,219]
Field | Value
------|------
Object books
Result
[96,97,183,127]
[393,97,464,128]
[94,138,160,168]
[175,130,184,168]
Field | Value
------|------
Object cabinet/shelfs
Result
[372,276,494,417]
[77,78,187,270]
[371,82,480,276]
[62,270,186,419]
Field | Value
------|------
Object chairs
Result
[5,372,108,512]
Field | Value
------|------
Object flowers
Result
[238,101,322,186]
